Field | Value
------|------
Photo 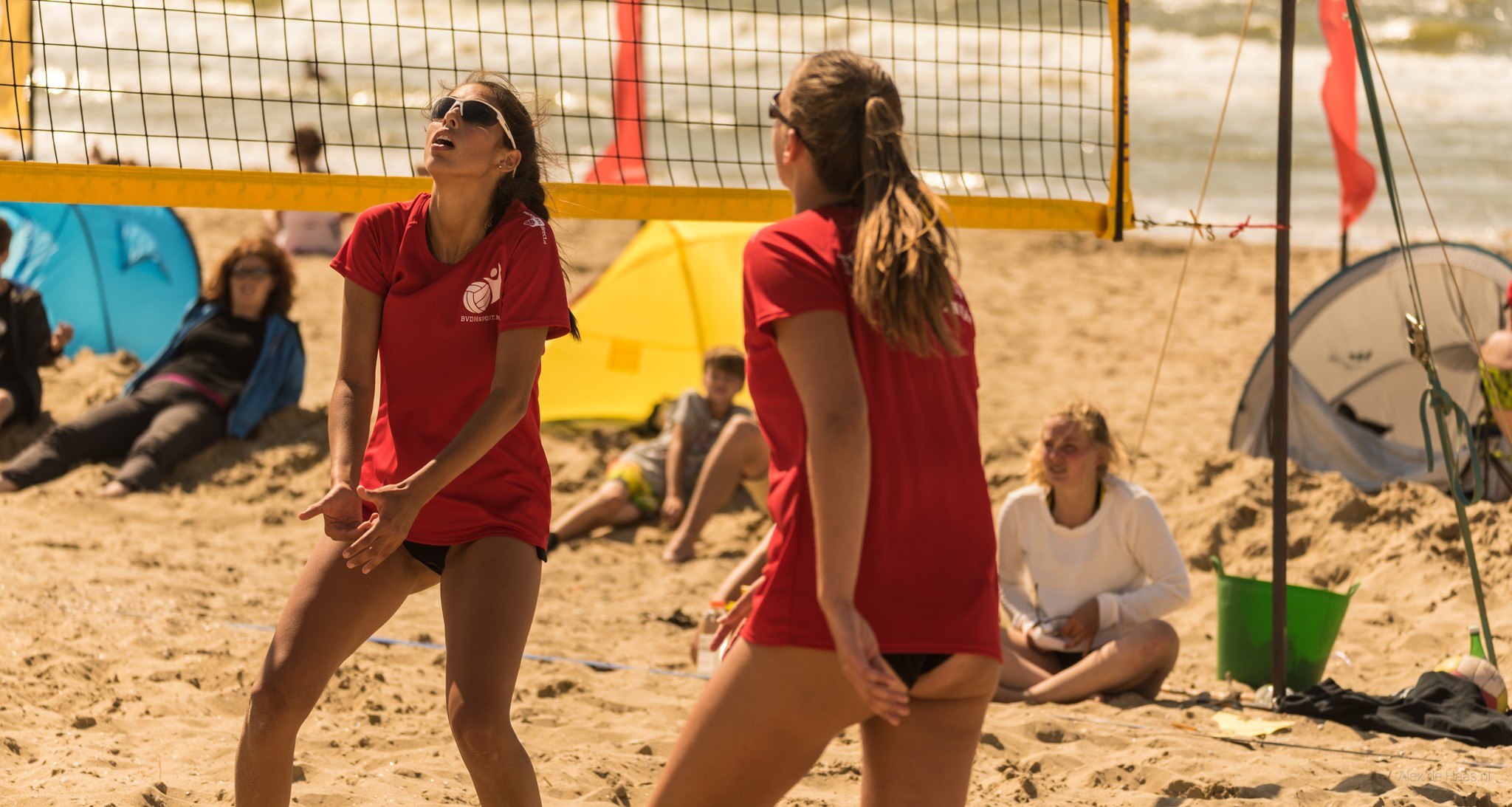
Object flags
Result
[1318,0,1379,232]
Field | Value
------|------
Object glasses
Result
[430,96,518,179]
[768,91,815,153]
[230,268,272,281]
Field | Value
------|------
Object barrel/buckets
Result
[1209,554,1361,692]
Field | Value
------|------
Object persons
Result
[654,47,1000,807]
[0,233,305,501]
[545,344,778,627]
[266,125,351,254]
[235,77,571,807]
[0,214,75,433]
[984,400,1191,711]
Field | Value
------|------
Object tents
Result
[0,200,203,373]
[535,218,774,426]
[1225,238,1511,513]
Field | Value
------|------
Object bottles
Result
[1468,625,1487,661]
[695,599,729,675]
